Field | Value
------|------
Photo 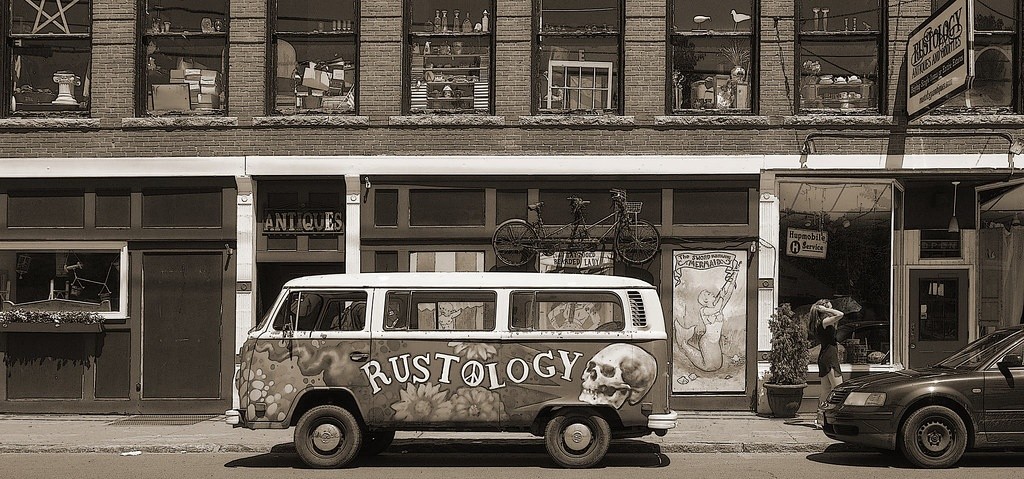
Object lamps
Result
[947,182,960,232]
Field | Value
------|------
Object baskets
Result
[624,201,642,213]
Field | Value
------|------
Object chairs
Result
[277,40,300,111]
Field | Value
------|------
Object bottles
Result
[453,9,459,31]
[434,11,441,33]
[442,11,448,32]
[482,9,489,32]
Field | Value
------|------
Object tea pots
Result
[847,76,860,80]
[834,77,846,81]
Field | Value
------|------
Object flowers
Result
[0,308,106,328]
[803,60,821,76]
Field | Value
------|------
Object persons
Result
[809,300,844,429]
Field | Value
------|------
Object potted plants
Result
[763,303,811,418]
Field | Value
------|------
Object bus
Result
[224,268,678,469]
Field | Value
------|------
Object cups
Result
[202,18,211,31]
[215,19,222,31]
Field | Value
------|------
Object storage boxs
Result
[150,67,221,112]
[300,61,357,112]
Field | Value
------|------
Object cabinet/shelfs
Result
[409,53,490,113]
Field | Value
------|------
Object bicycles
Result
[490,188,661,267]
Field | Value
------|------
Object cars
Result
[815,323,1024,470]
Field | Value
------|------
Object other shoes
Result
[814,419,823,429]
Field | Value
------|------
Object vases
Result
[805,76,819,85]
[0,324,103,333]
[731,66,746,82]
